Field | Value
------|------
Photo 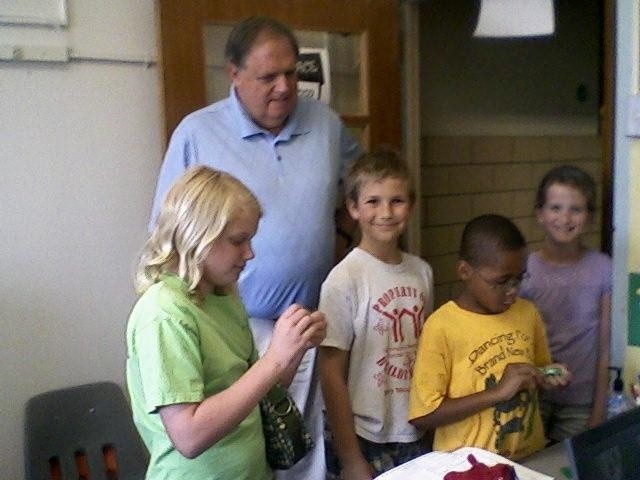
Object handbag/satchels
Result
[257,378,317,469]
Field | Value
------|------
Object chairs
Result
[21,380,149,480]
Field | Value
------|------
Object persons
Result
[145,15,368,480]
[511,160,616,447]
[122,162,330,480]
[311,147,439,480]
[405,211,576,464]
